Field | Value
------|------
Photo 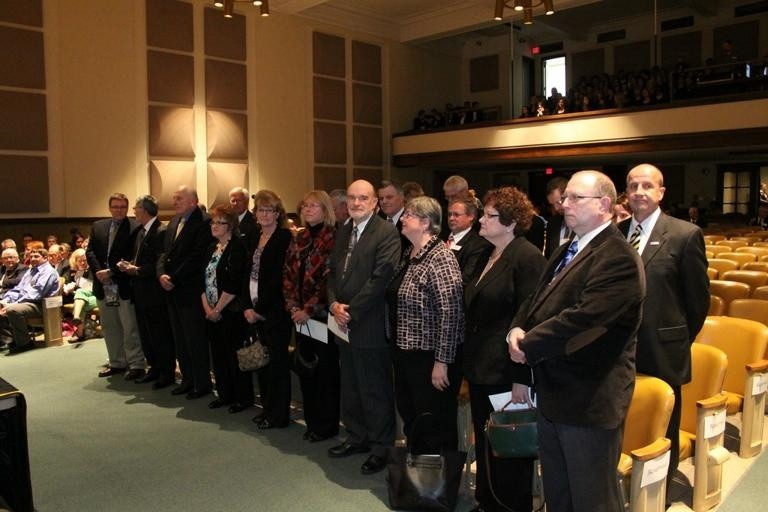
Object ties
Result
[446,236,453,247]
[553,241,578,275]
[111,222,119,241]
[134,228,145,266]
[343,226,358,272]
[628,224,642,252]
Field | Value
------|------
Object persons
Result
[87,163,711,512]
[0,228,98,354]
[412,100,483,129]
[520,40,742,118]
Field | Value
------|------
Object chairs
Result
[64,303,103,338]
[27,277,66,346]
[458,225,768,512]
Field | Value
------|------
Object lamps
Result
[213,0,270,18]
[494,0,554,25]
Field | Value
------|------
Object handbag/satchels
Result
[388,447,467,512]
[487,408,539,458]
[75,318,98,340]
[236,338,270,370]
[292,337,327,376]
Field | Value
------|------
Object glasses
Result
[482,213,500,219]
[209,220,228,226]
[559,194,602,204]
[258,208,277,214]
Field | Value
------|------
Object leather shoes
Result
[303,426,340,443]
[361,454,391,474]
[253,413,289,428]
[329,439,370,458]
[98,364,254,413]
